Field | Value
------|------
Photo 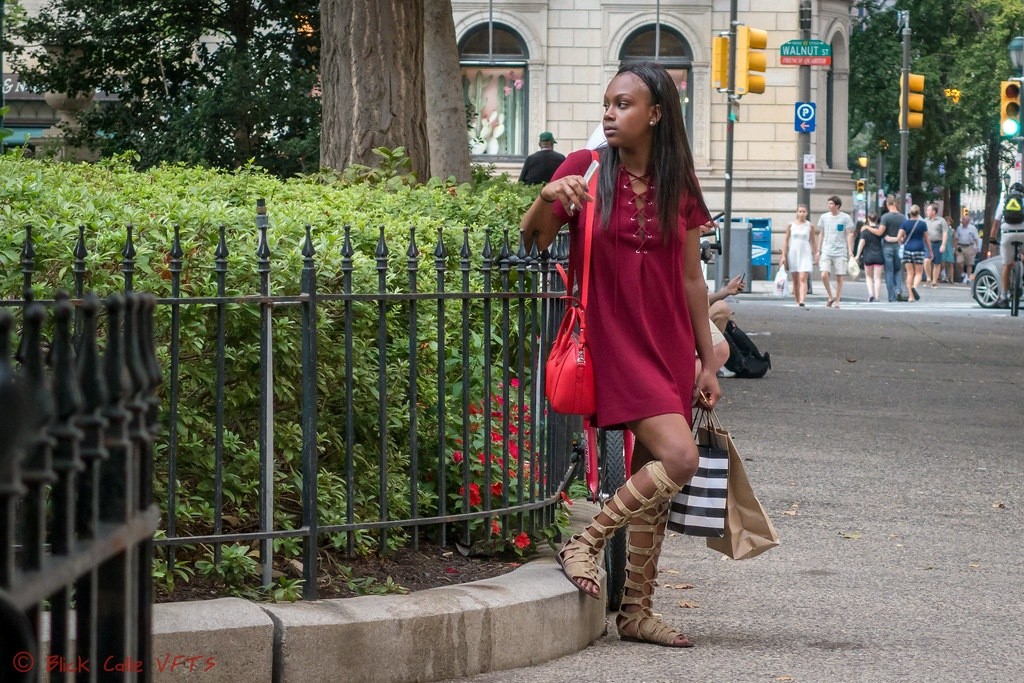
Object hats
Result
[1011,183,1024,193]
[540,132,557,144]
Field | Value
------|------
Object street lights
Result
[859,151,869,221]
[1001,81,1022,138]
[1008,36,1024,188]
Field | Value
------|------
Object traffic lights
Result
[963,207,969,216]
[713,36,730,88]
[857,180,864,201]
[898,71,926,126]
[735,25,767,95]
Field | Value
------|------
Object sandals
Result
[557,461,684,601]
[615,502,694,647]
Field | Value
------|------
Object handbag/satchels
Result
[848,256,861,279]
[773,264,788,296]
[544,332,596,415]
[701,407,779,561]
[667,404,728,538]
[898,244,904,259]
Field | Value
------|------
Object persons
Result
[854,182,1024,309]
[695,318,731,387]
[520,63,722,648]
[706,275,746,377]
[519,132,566,184]
[816,196,855,308]
[779,204,818,306]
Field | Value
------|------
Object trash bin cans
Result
[715,216,771,282]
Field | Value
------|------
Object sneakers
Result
[998,299,1009,308]
[924,273,971,288]
[869,288,920,302]
[716,366,736,378]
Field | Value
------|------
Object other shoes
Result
[826,298,835,308]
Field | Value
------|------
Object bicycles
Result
[989,238,1023,315]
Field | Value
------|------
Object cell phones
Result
[711,211,725,222]
[737,272,745,292]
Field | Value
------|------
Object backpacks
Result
[724,319,772,378]
[1004,194,1024,223]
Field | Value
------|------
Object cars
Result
[971,255,1024,308]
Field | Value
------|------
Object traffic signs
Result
[803,155,818,188]
[781,39,833,67]
